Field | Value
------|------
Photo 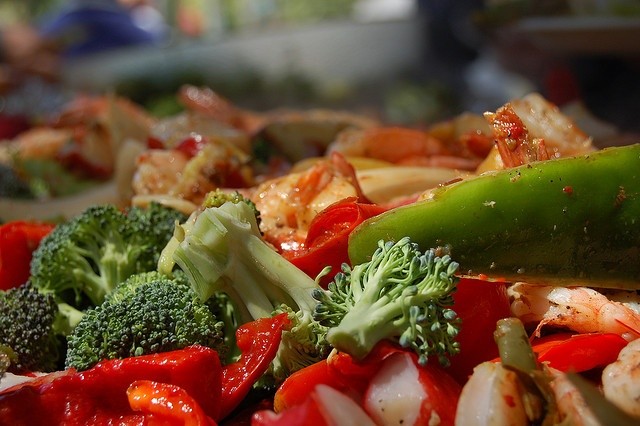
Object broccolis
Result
[1,287,64,370]
[310,235,463,363]
[175,209,336,368]
[27,204,173,309]
[62,274,228,370]
[156,189,262,278]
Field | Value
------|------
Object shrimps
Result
[506,282,640,343]
[601,338,640,420]
[483,91,600,169]
[59,85,250,140]
[14,126,114,182]
[326,126,481,171]
[250,160,359,252]
[131,149,249,210]
[453,360,600,426]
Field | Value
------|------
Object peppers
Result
[346,146,639,283]
